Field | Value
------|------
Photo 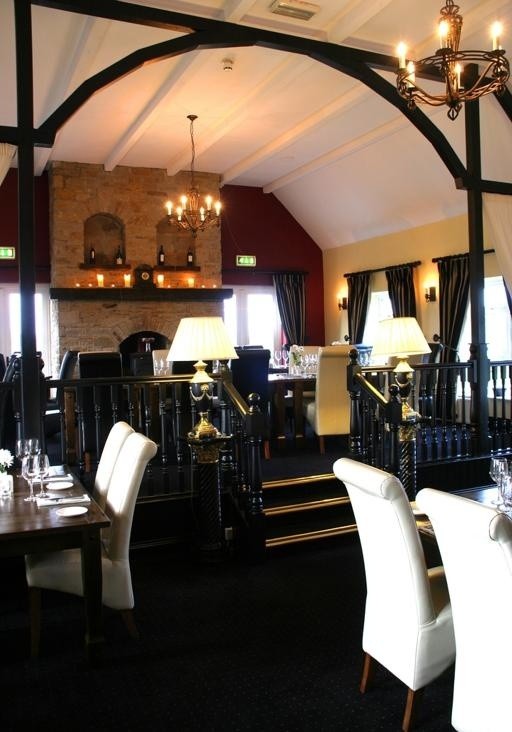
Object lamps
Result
[164,315,240,440]
[425,286,436,303]
[338,297,347,310]
[369,315,434,422]
[391,0,511,122]
[163,114,222,238]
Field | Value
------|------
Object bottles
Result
[90,242,96,263]
[187,245,193,267]
[116,245,123,265]
[158,244,165,265]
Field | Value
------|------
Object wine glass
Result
[15,437,49,501]
[273,348,318,379]
[488,456,511,512]
[154,359,169,377]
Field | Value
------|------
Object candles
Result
[187,277,195,289]
[124,273,131,287]
[157,273,165,288]
[96,273,105,288]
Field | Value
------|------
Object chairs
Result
[90,421,135,539]
[332,456,456,732]
[23,433,162,661]
[0,342,446,462]
[415,486,512,732]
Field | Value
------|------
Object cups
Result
[360,353,368,367]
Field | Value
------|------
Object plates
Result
[47,481,74,491]
[56,506,88,517]
